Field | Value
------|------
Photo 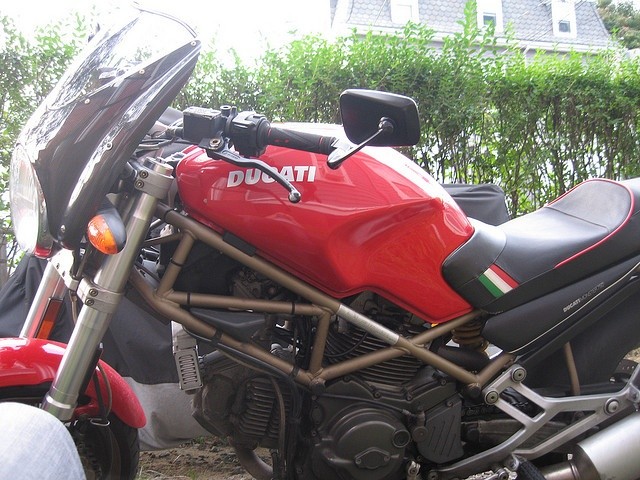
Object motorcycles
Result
[0,7,640,480]
